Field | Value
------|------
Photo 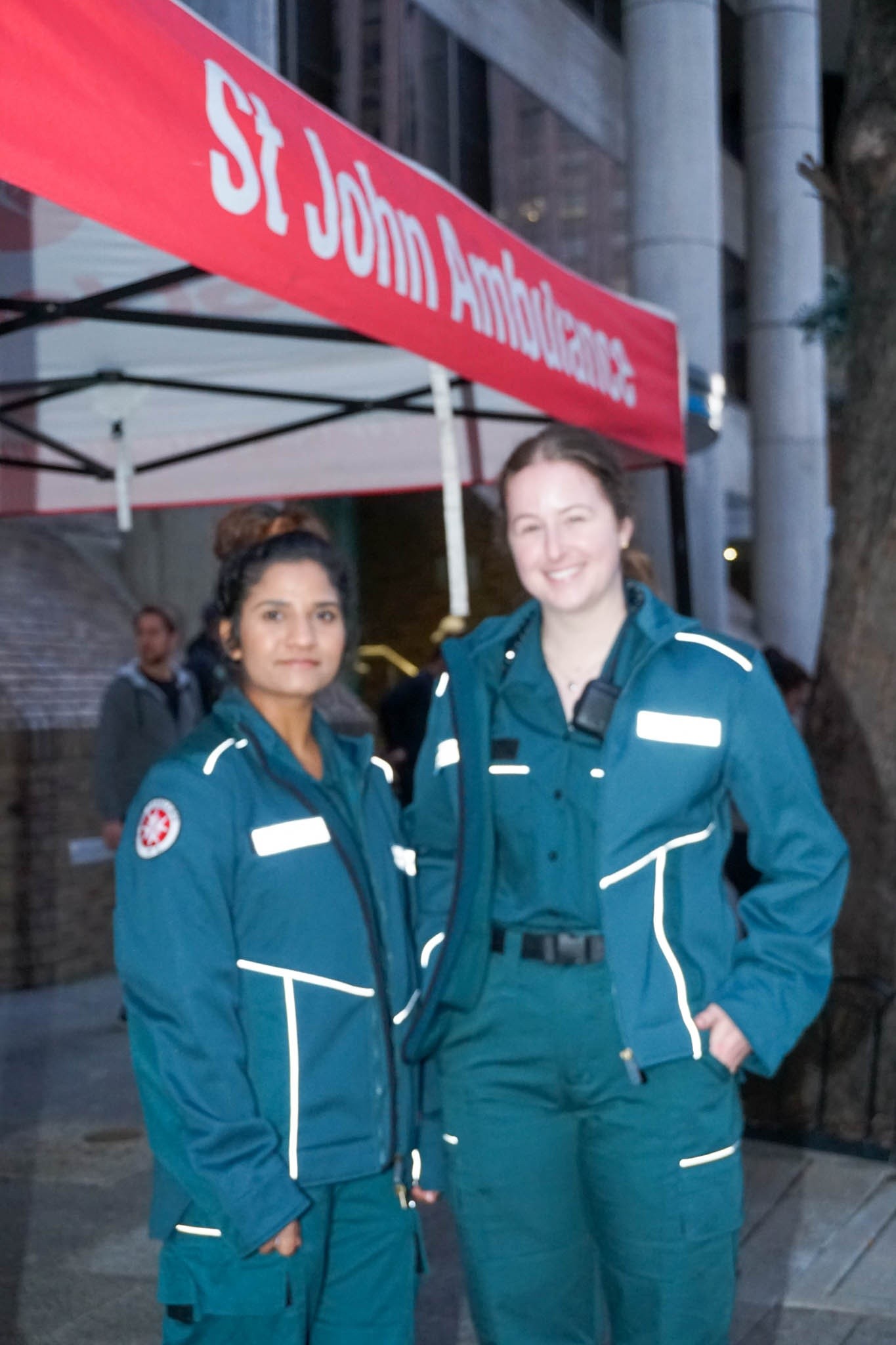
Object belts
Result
[492,926,603,965]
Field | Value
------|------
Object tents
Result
[2,0,683,602]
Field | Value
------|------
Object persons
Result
[724,647,812,939]
[113,528,449,1345]
[186,499,386,761]
[390,651,449,806]
[404,429,850,1345]
[92,604,202,1022]
